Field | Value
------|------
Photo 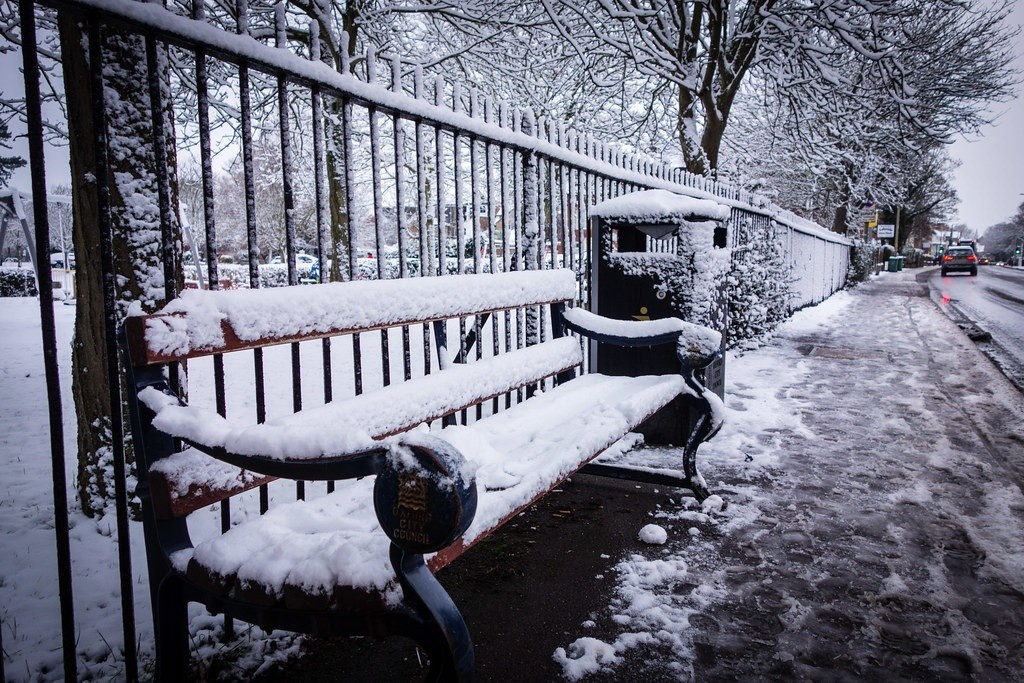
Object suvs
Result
[941,246,978,277]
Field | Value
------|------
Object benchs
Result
[118,268,727,682]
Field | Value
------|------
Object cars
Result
[957,239,977,254]
[996,261,1004,266]
[270,253,319,265]
[979,258,988,265]
[50,248,76,270]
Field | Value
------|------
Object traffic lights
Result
[1016,246,1021,254]
[939,248,944,253]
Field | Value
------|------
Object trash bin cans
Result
[590,189,730,450]
